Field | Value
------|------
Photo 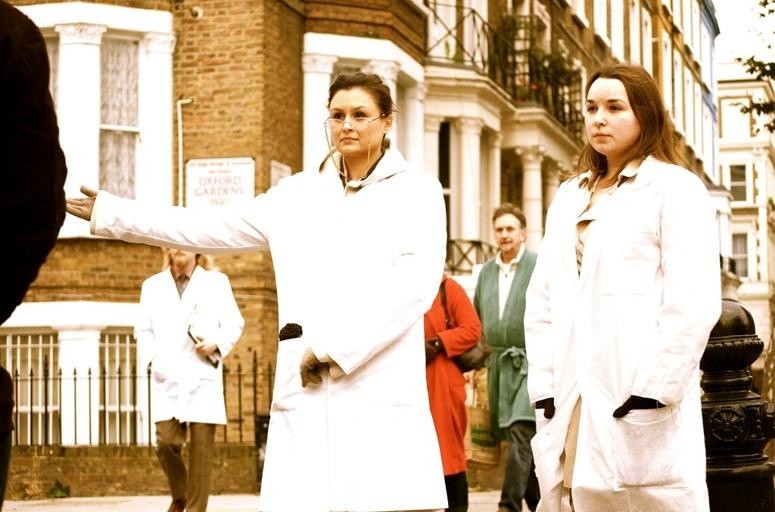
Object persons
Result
[137,246,246,512]
[65,72,449,512]
[475,202,537,512]
[522,63,722,512]
[0,0,68,512]
[424,273,482,512]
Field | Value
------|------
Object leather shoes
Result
[167,498,185,512]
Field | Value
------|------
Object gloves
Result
[535,398,555,419]
[65,185,97,220]
[613,395,665,417]
[425,336,443,362]
[300,350,322,387]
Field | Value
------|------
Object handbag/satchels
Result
[463,380,502,471]
[439,278,491,373]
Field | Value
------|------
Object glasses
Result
[322,114,383,128]
